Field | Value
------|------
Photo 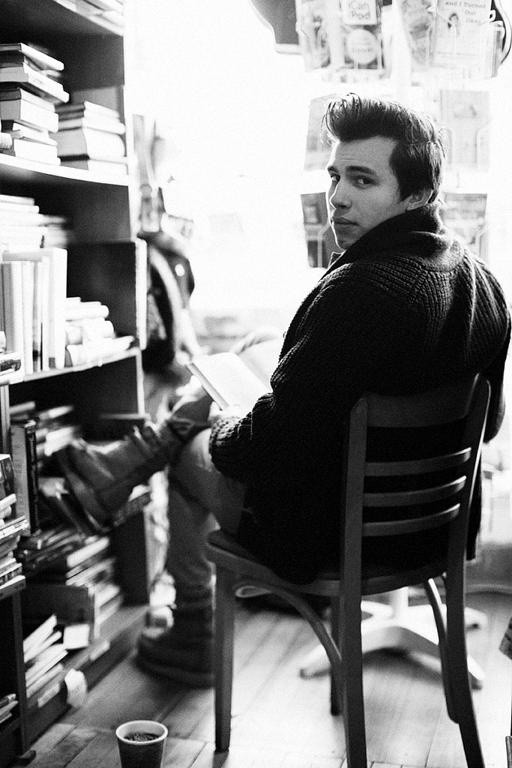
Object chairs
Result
[206,375,491,768]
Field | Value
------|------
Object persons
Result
[56,94,512,688]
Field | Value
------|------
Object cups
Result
[116,720,168,768]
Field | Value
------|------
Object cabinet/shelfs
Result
[0,0,150,767]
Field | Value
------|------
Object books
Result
[0,382,56,584]
[0,192,68,376]
[55,536,127,624]
[0,43,70,167]
[187,341,284,416]
[20,526,79,558]
[24,615,67,711]
[66,295,136,367]
[58,622,92,652]
[56,101,128,174]
[1,692,19,724]
[38,405,82,458]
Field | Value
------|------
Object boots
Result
[138,605,216,683]
[53,425,165,536]
